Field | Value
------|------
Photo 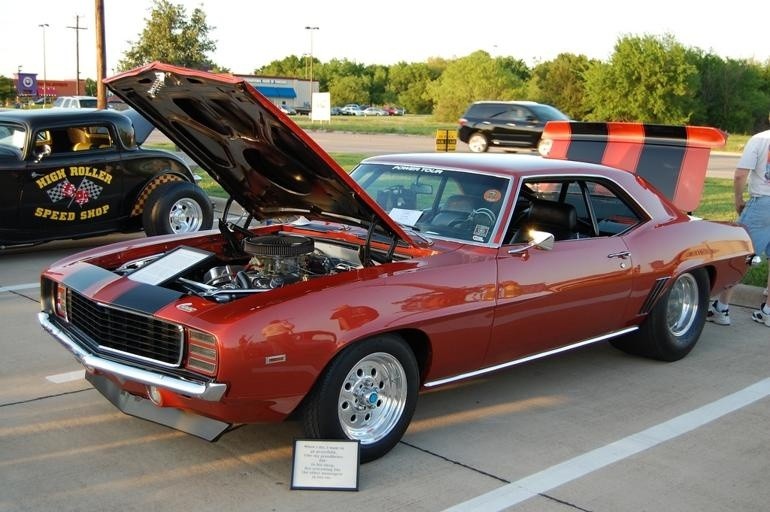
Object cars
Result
[279,103,404,117]
[52,95,115,110]
[0,108,216,253]
[35,97,51,105]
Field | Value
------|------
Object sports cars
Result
[37,63,759,462]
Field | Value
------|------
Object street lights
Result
[38,23,50,107]
[305,27,321,107]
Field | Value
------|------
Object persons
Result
[701,113,770,328]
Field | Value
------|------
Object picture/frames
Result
[290,437,361,491]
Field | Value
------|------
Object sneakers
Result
[705,300,731,325]
[751,303,770,327]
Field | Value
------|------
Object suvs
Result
[457,100,580,154]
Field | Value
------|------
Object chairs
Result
[511,200,580,245]
[431,195,483,232]
